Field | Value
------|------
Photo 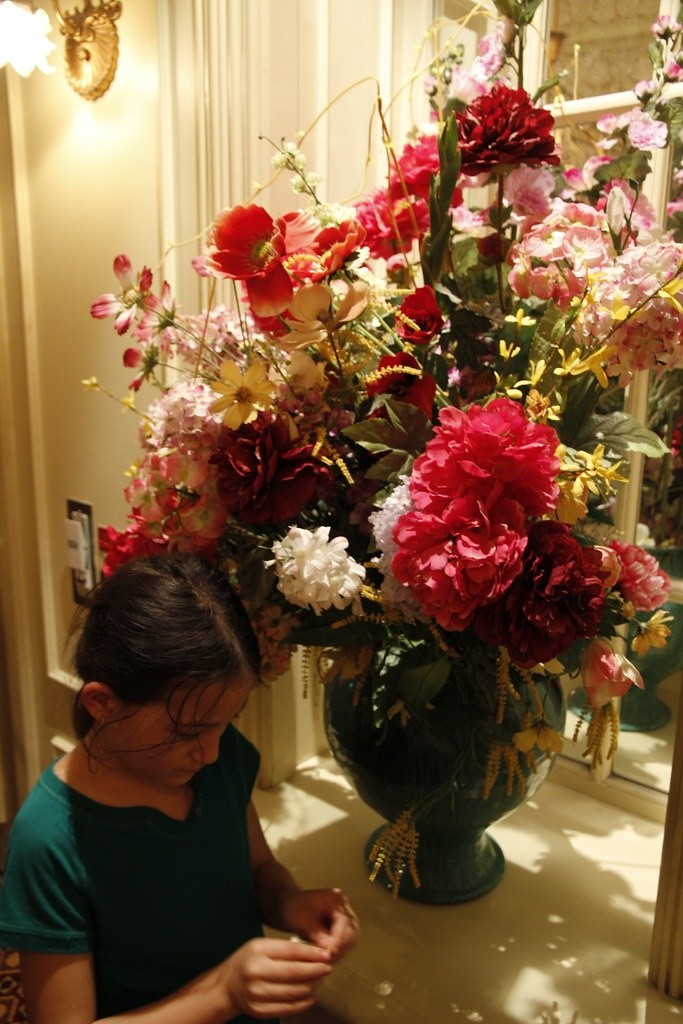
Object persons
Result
[0,551,359,1024]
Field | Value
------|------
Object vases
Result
[568,546,682,733]
[323,642,565,907]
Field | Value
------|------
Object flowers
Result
[574,370,682,545]
[83,0,683,811]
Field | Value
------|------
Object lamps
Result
[0,0,122,103]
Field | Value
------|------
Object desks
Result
[253,786,683,1024]
